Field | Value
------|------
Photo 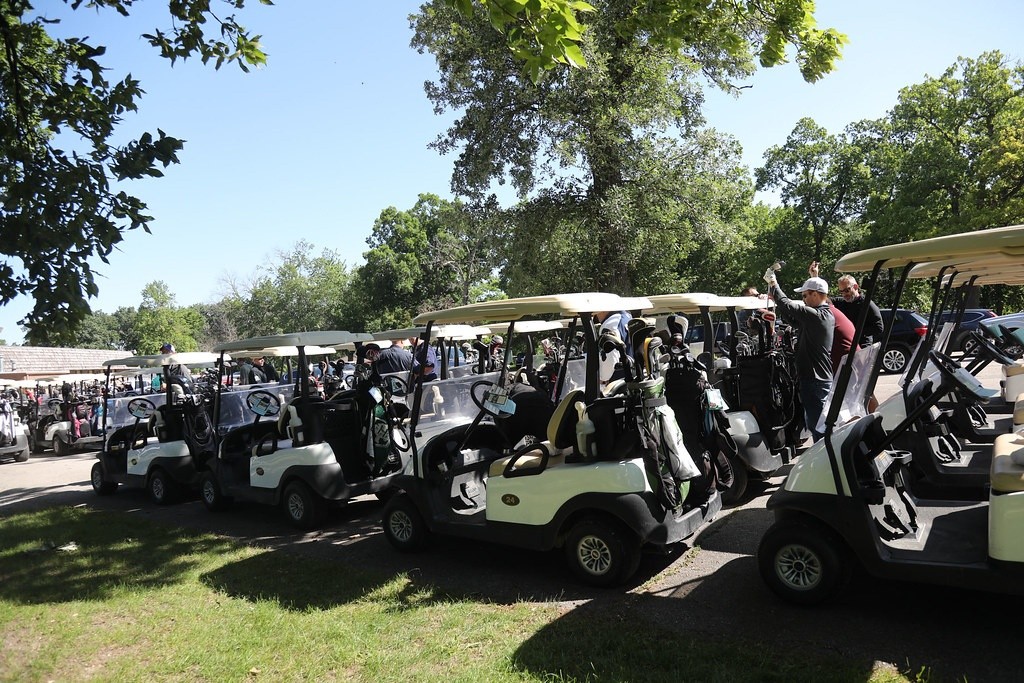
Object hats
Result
[794,277,828,294]
[160,344,175,353]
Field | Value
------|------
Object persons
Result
[825,298,864,427]
[0,302,690,448]
[736,287,776,339]
[762,267,836,444]
[808,261,884,418]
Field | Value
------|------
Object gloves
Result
[763,268,777,287]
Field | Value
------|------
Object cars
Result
[879,308,934,374]
[921,308,997,356]
[977,309,1024,361]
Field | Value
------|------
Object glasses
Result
[840,287,851,293]
[802,294,808,298]
